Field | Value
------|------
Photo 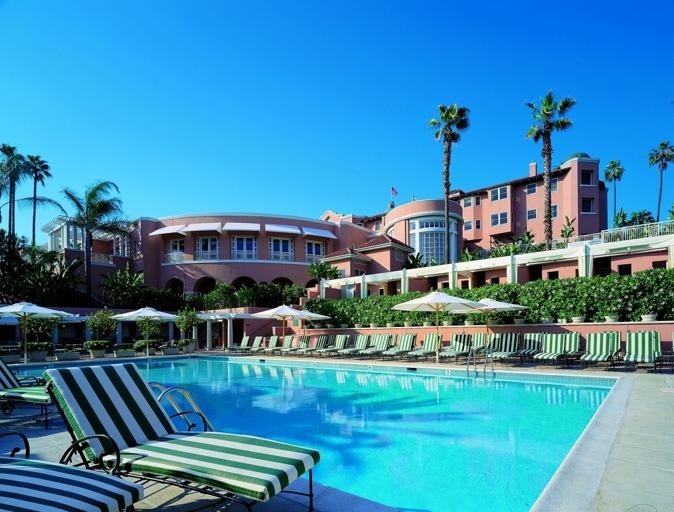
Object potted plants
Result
[22,338,198,362]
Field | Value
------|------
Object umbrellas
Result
[252,305,333,348]
[252,369,328,414]
[111,306,180,356]
[390,291,531,358]
[0,300,78,364]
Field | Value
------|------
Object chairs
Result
[0,428,146,510]
[224,333,470,366]
[0,355,60,428]
[42,362,320,511]
[469,331,662,368]
